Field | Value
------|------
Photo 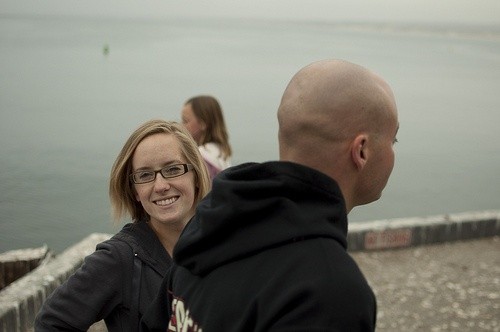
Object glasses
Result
[128,164,193,184]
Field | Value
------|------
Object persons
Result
[180,95,232,183]
[166,59,400,332]
[34,119,211,332]
